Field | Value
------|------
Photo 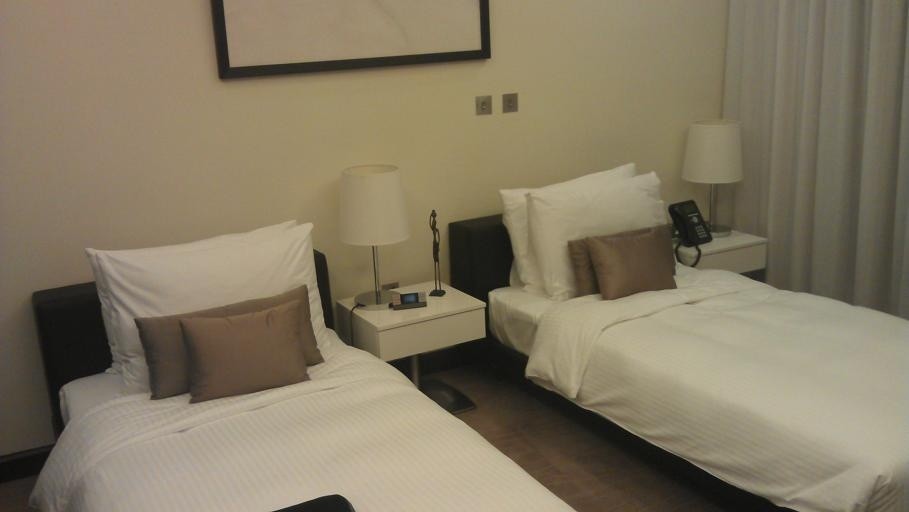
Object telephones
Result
[668,199,713,269]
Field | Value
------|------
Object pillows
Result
[177,299,313,404]
[133,285,325,400]
[86,218,299,375]
[95,223,330,397]
[498,158,683,302]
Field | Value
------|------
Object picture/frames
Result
[208,1,492,80]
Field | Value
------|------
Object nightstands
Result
[670,227,768,280]
[338,279,488,414]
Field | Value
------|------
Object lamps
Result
[681,122,744,239]
[338,163,414,311]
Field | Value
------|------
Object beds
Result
[27,250,586,512]
[449,194,909,512]
